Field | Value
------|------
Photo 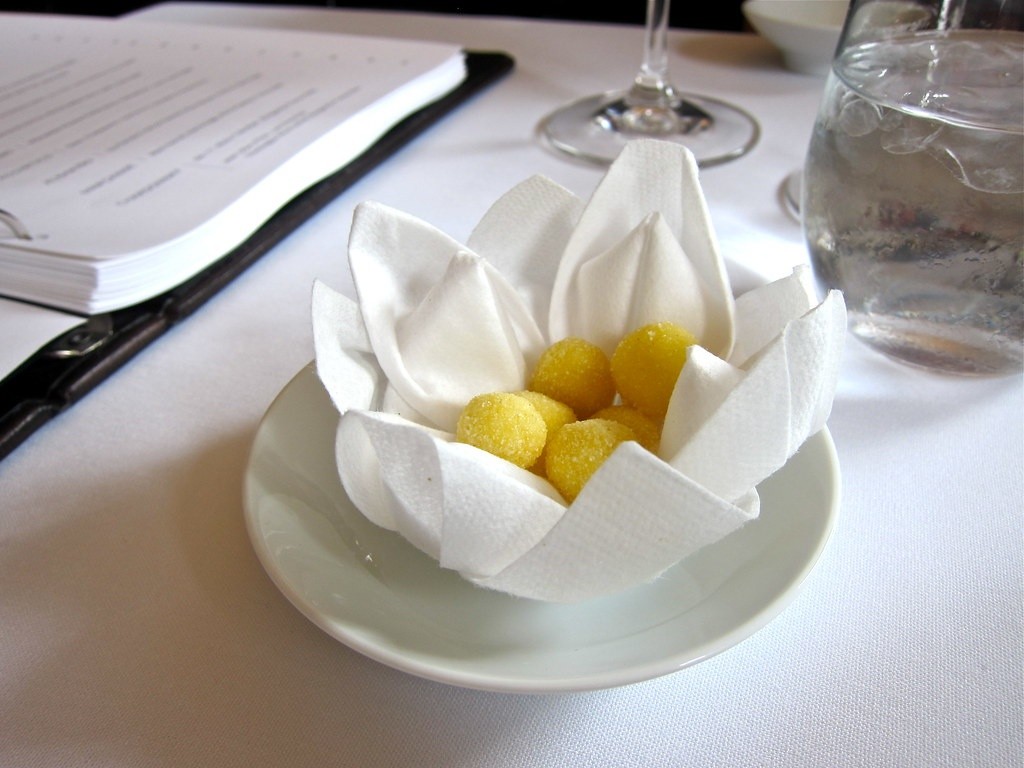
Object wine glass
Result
[532,0,762,171]
[773,0,970,230]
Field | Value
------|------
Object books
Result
[0,12,518,463]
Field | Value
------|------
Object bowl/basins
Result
[741,0,935,76]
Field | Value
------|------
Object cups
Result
[799,0,1024,381]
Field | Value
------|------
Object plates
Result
[242,357,843,696]
